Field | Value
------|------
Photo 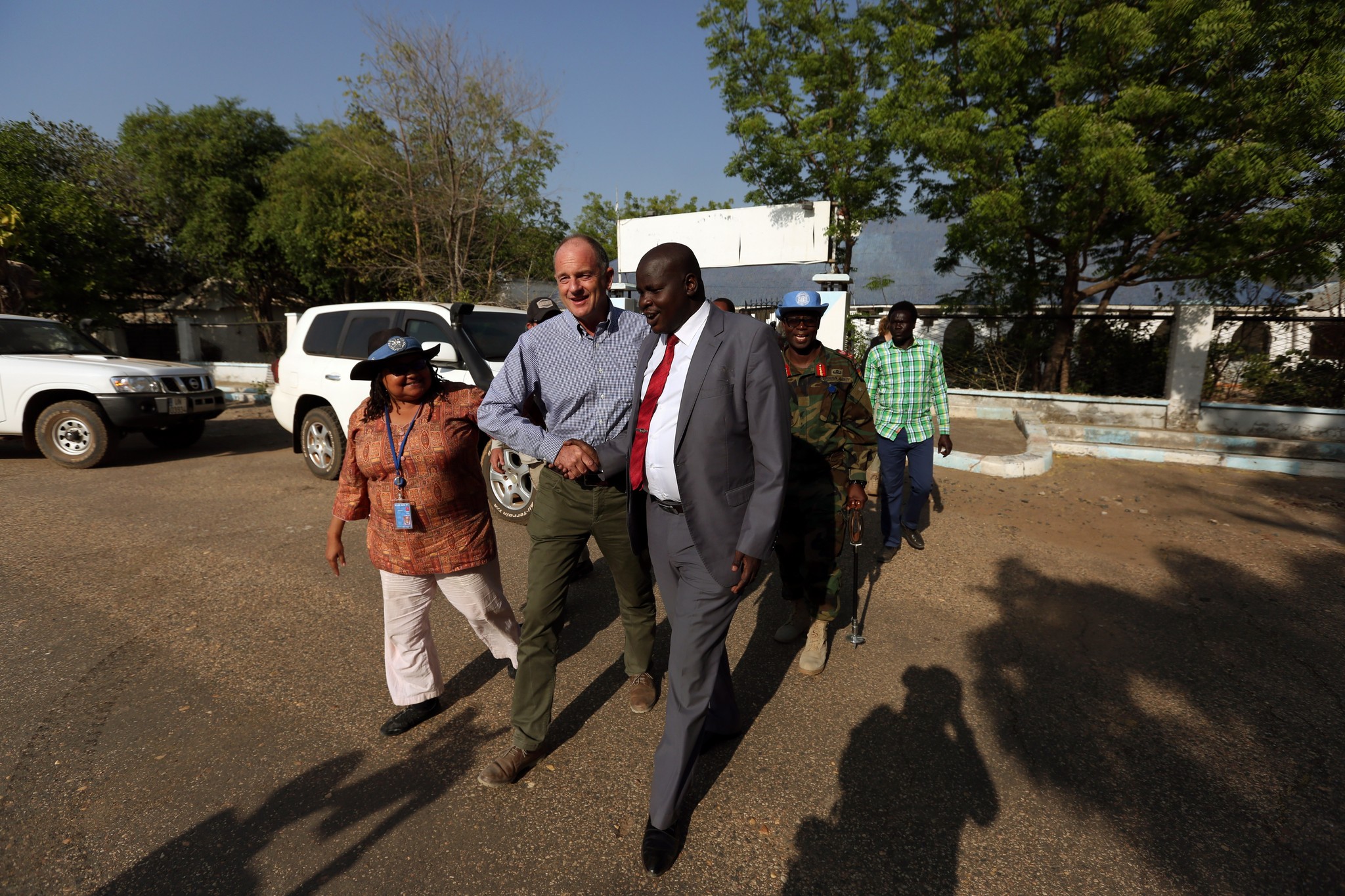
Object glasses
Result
[784,317,820,327]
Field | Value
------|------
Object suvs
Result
[1,313,228,468]
[269,297,566,524]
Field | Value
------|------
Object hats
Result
[350,335,441,382]
[775,290,829,321]
[526,297,562,322]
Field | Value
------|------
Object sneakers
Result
[379,697,442,736]
[507,659,517,680]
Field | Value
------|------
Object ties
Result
[628,334,680,492]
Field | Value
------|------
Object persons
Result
[768,288,879,678]
[545,241,797,880]
[324,327,524,737]
[476,234,664,789]
[864,301,954,565]
[712,297,736,314]
[489,297,565,475]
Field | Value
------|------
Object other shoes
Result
[567,558,594,585]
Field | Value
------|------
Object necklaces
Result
[861,315,893,380]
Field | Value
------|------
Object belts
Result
[650,497,684,516]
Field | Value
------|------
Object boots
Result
[774,600,811,643]
[797,617,828,675]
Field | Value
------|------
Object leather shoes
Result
[629,665,656,713]
[477,744,546,788]
[876,545,898,563]
[640,813,681,878]
[899,514,925,549]
